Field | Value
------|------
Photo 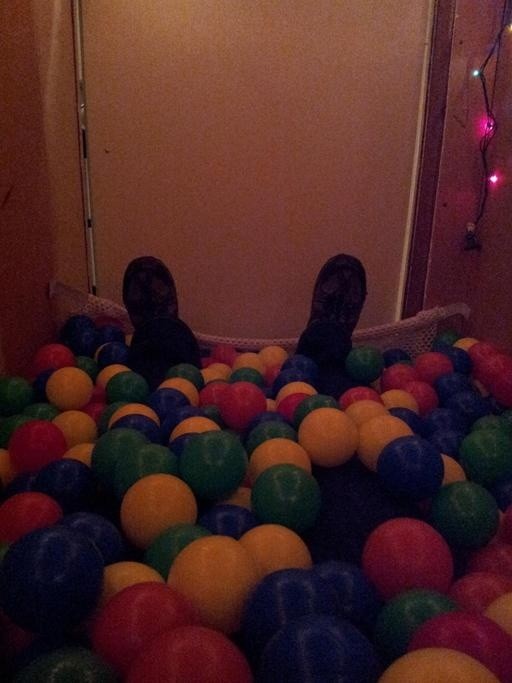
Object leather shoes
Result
[123,257,178,337]
[309,253,367,349]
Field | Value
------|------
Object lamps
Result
[473,69,481,76]
[486,121,495,128]
[490,175,498,182]
[506,23,512,33]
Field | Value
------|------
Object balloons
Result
[428,409,466,440]
[327,463,390,538]
[435,332,456,347]
[202,356,210,367]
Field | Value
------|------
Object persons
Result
[122,253,369,362]
